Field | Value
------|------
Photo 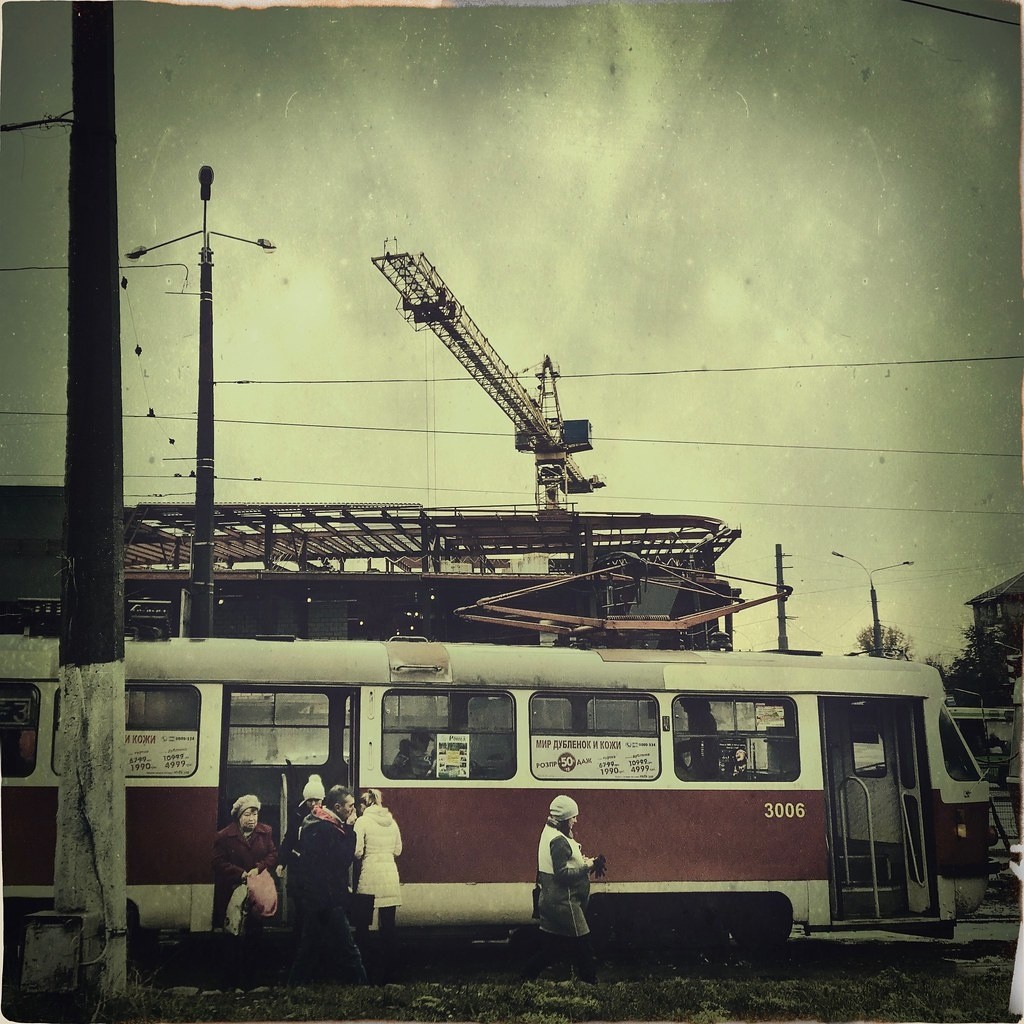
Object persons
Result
[674,696,719,780]
[394,728,481,779]
[289,784,372,988]
[209,794,280,930]
[353,788,403,952]
[276,774,327,946]
[521,793,606,982]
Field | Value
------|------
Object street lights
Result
[829,551,916,659]
[120,165,277,638]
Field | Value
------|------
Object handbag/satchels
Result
[223,868,279,937]
[532,888,542,920]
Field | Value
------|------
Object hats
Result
[302,774,325,800]
[231,794,261,818]
[550,795,579,821]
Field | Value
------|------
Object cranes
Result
[369,234,607,515]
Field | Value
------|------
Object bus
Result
[1006,672,1024,907]
[0,535,1000,979]
[758,704,1014,802]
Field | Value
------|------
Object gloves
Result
[590,854,607,878]
